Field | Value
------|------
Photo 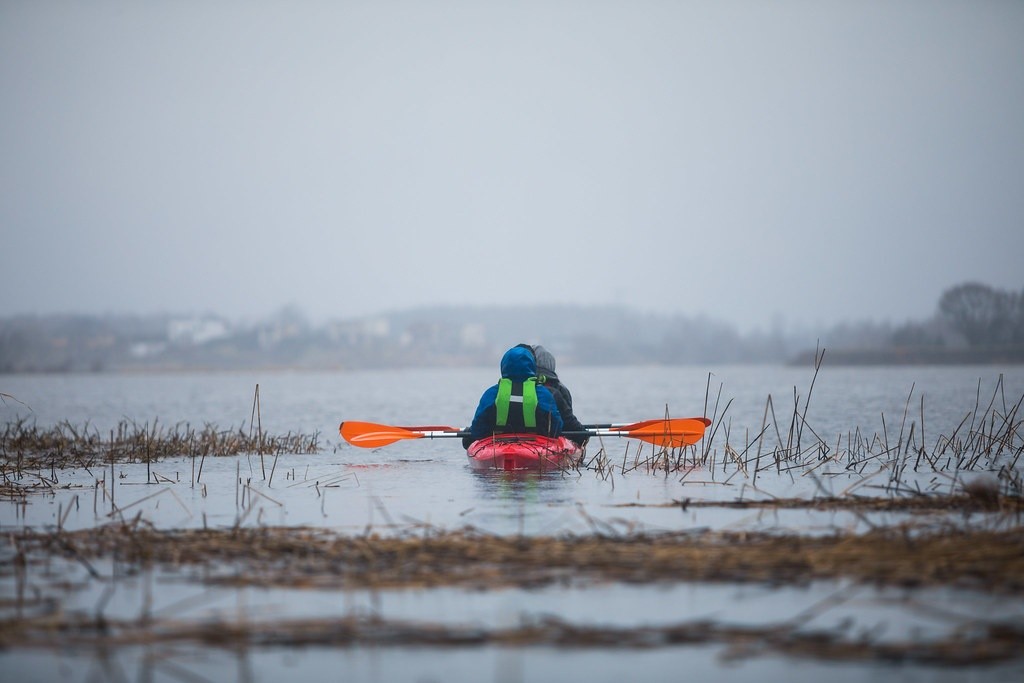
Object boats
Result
[467,432,589,472]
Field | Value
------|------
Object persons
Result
[461,342,590,450]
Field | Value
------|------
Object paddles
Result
[338,417,714,449]
